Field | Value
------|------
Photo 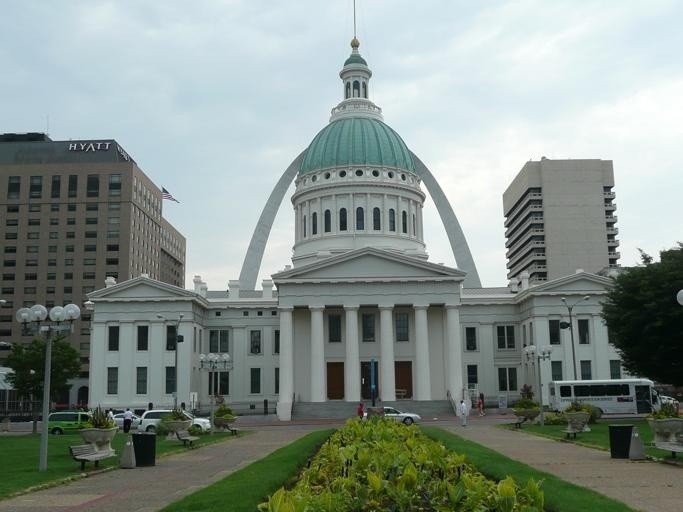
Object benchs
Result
[512,417,525,428]
[176,431,200,446]
[69,444,113,472]
[225,424,239,435]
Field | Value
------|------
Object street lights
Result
[157,312,183,409]
[524,345,552,426]
[16,303,79,473]
[560,297,590,379]
[197,352,229,430]
[0,299,8,307]
[676,288,682,307]
[0,342,12,352]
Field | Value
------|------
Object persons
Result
[107,408,114,421]
[358,402,365,419]
[459,400,467,426]
[478,397,484,416]
[123,409,134,434]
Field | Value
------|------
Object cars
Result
[110,413,140,430]
[362,407,420,426]
[661,396,680,406]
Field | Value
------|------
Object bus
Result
[547,380,660,419]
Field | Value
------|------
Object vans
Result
[138,410,211,434]
[47,412,95,436]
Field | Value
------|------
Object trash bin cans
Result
[131,432,155,466]
[608,424,634,458]
